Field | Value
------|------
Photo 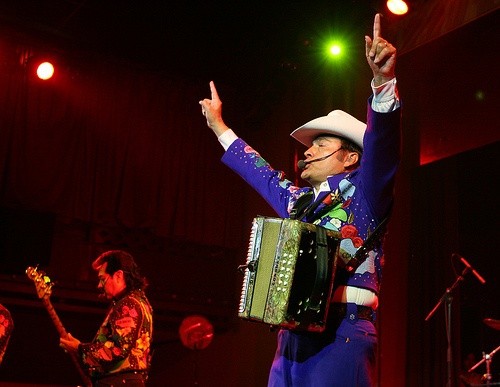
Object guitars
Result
[25,266,95,387]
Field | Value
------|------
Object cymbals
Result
[483,318,500,330]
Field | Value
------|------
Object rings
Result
[381,41,388,46]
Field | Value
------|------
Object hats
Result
[290,110,367,149]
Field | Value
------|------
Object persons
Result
[60,249,153,387]
[199,14,401,387]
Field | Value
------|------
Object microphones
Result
[452,252,486,284]
[237,261,257,275]
[297,143,347,168]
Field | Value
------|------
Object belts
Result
[328,302,374,322]
